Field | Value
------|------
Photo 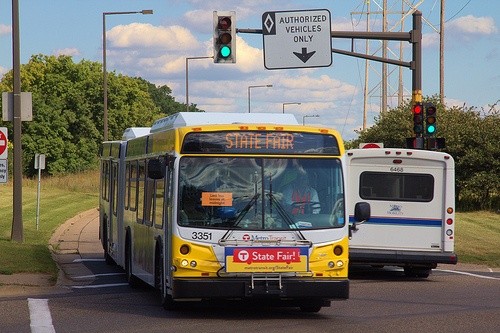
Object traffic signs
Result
[262,9,333,71]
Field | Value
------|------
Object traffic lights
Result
[406,136,424,149]
[412,105,425,134]
[217,15,234,61]
[426,105,436,135]
[428,137,446,150]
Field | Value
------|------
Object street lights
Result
[302,115,319,127]
[283,102,302,114]
[248,85,272,113]
[103,9,154,139]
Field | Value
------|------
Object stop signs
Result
[0,127,8,159]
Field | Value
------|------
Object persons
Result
[281,169,321,214]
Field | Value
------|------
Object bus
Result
[346,149,460,278]
[98,112,349,314]
